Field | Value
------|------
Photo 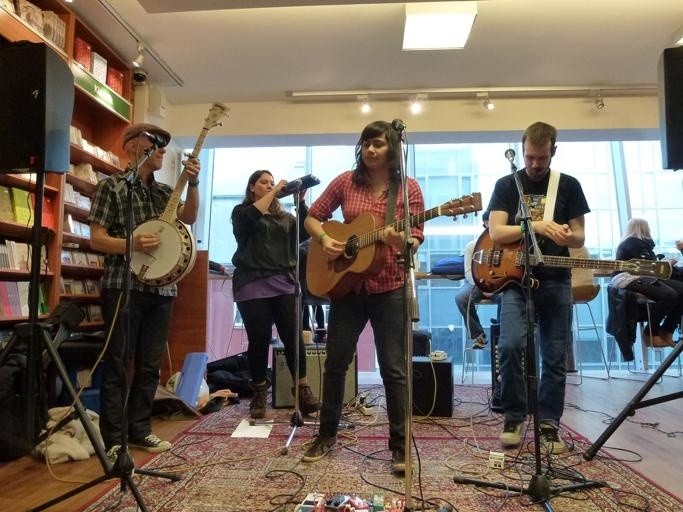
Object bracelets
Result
[519,222,527,235]
[316,233,325,241]
[188,179,199,187]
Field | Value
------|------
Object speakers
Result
[413,356,453,417]
[271,346,358,409]
[657,45,683,171]
[0,40,76,175]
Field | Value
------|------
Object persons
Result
[231,170,323,418]
[677,240,683,256]
[301,121,425,475]
[455,208,502,349]
[611,218,683,348]
[487,122,590,455]
[88,123,200,466]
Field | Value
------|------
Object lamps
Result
[290,84,660,116]
[401,1,477,51]
[95,0,185,86]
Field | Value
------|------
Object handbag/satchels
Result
[430,256,464,275]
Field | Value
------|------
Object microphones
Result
[144,131,167,148]
[392,118,406,131]
[295,178,303,193]
[505,149,516,162]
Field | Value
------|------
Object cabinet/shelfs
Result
[160,248,279,388]
[56,12,136,330]
[0,0,73,351]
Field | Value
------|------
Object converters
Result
[488,450,506,469]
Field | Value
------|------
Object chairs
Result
[565,283,610,385]
[461,299,497,385]
[606,282,681,385]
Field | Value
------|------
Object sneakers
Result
[499,421,522,445]
[247,375,272,418]
[391,450,414,473]
[313,327,327,342]
[291,383,322,414]
[105,445,135,478]
[303,433,337,462]
[540,421,565,454]
[128,434,172,453]
[472,334,488,349]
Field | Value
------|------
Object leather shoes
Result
[659,332,675,347]
[644,335,669,347]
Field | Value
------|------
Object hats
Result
[122,123,171,151]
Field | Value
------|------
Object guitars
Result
[471,223,672,295]
[306,191,481,300]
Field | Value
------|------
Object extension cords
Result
[356,401,374,416]
[430,351,447,361]
[323,495,351,512]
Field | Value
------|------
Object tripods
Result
[0,173,114,477]
[249,190,356,456]
[28,145,183,512]
[453,163,609,512]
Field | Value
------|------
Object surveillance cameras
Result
[133,68,148,83]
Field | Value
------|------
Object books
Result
[1,0,124,323]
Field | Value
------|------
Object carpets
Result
[74,383,683,512]
